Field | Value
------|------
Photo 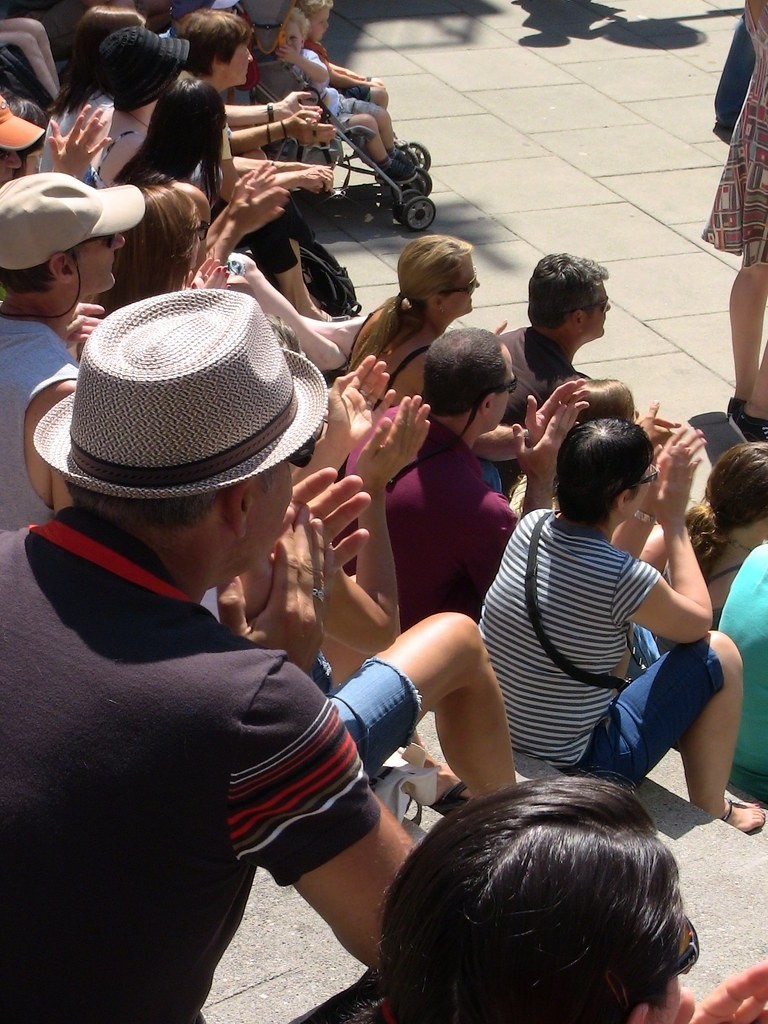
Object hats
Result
[0,96,46,151]
[32,286,333,502]
[95,25,192,111]
[0,170,147,270]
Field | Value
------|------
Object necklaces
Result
[128,111,149,129]
[728,535,753,551]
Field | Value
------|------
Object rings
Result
[85,144,91,151]
[687,474,693,480]
[358,389,371,398]
[312,129,318,138]
[311,588,325,604]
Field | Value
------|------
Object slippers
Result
[438,778,474,805]
[720,796,766,836]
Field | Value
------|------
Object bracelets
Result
[266,102,276,123]
[265,124,275,146]
[633,509,657,526]
[280,120,290,139]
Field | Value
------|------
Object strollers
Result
[235,0,436,232]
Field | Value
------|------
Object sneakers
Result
[379,151,420,185]
[727,396,768,444]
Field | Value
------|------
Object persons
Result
[0,0,768,834]
[700,1,768,444]
[378,778,768,1024]
[0,287,419,1024]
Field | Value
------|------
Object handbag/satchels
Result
[296,248,363,318]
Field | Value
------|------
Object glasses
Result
[83,231,118,249]
[188,221,210,240]
[443,275,478,297]
[585,295,611,314]
[635,464,660,486]
